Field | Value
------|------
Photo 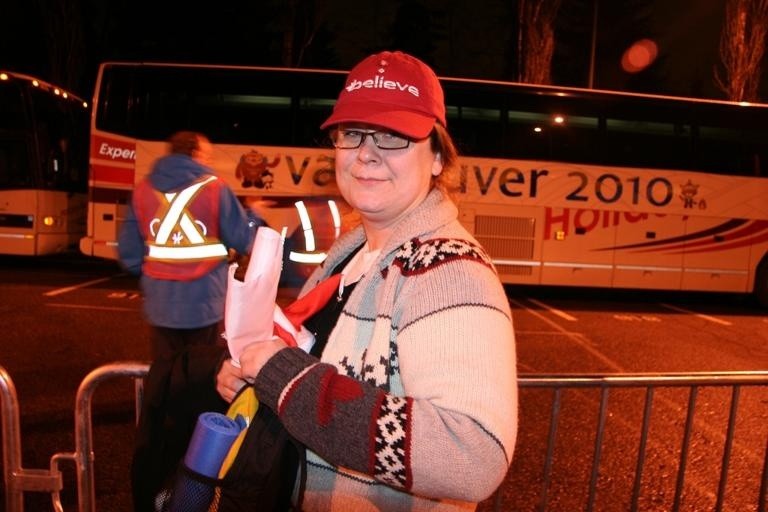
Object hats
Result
[318,49,450,140]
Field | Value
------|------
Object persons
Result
[115,130,266,512]
[212,50,521,512]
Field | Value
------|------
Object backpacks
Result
[134,330,309,512]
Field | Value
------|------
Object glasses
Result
[327,127,413,151]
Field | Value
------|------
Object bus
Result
[76,57,768,312]
[0,69,89,273]
